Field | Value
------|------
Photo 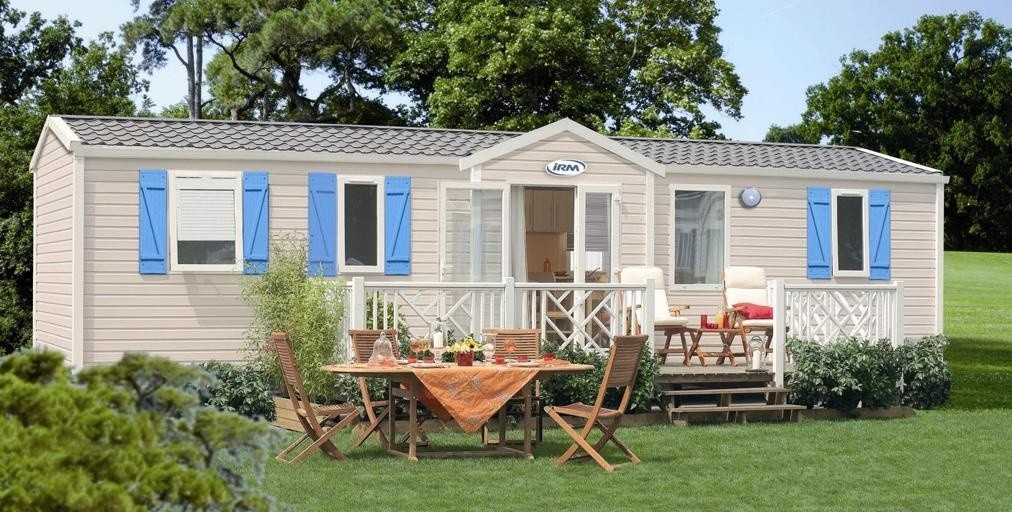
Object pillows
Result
[731,301,774,320]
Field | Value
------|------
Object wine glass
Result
[503,339,517,367]
[409,336,431,365]
[481,333,497,364]
[428,320,448,365]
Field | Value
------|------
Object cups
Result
[700,312,732,330]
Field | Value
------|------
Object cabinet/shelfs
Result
[522,186,575,234]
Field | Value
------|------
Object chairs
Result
[617,263,690,366]
[718,265,790,366]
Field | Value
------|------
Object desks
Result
[679,326,741,366]
